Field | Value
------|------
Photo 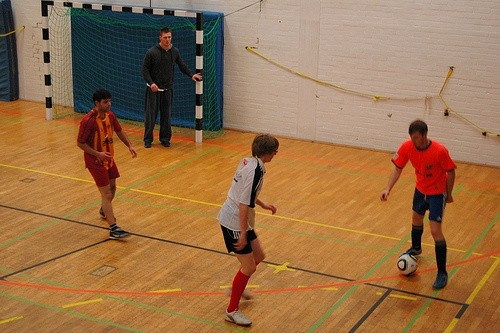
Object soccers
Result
[396,253,418,275]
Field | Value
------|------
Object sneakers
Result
[241,288,254,300]
[224,309,253,326]
[161,142,170,147]
[400,247,422,259]
[99,207,116,222]
[108,228,129,239]
[144,141,152,148]
[432,272,448,288]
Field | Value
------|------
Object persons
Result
[380,120,458,289]
[215,133,279,326]
[140,29,203,148]
[75,93,137,240]
